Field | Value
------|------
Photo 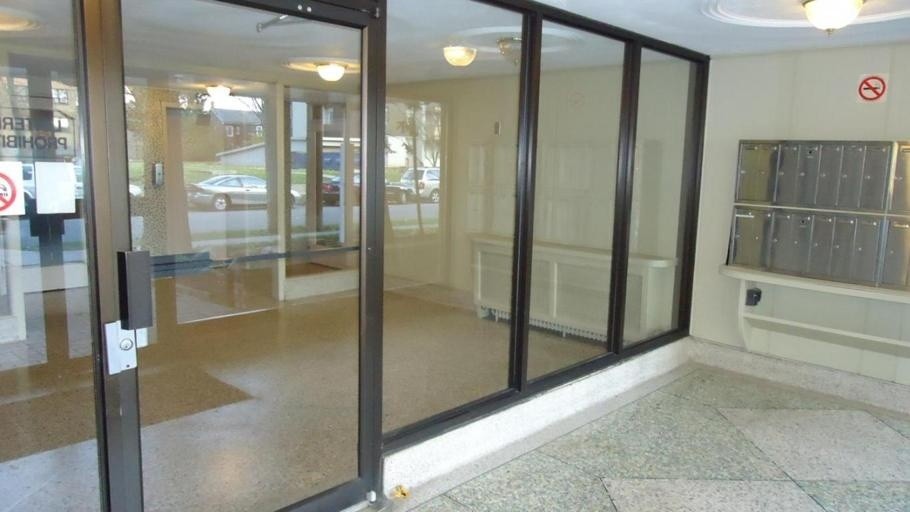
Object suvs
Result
[399,165,440,204]
[71,164,142,216]
[18,163,36,220]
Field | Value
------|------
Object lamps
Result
[496,37,522,65]
[316,63,346,82]
[206,86,230,100]
[443,46,477,66]
[802,0,863,30]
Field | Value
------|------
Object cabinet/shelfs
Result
[469,232,679,351]
[724,140,910,291]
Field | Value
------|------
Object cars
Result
[181,170,301,213]
[317,168,411,207]
